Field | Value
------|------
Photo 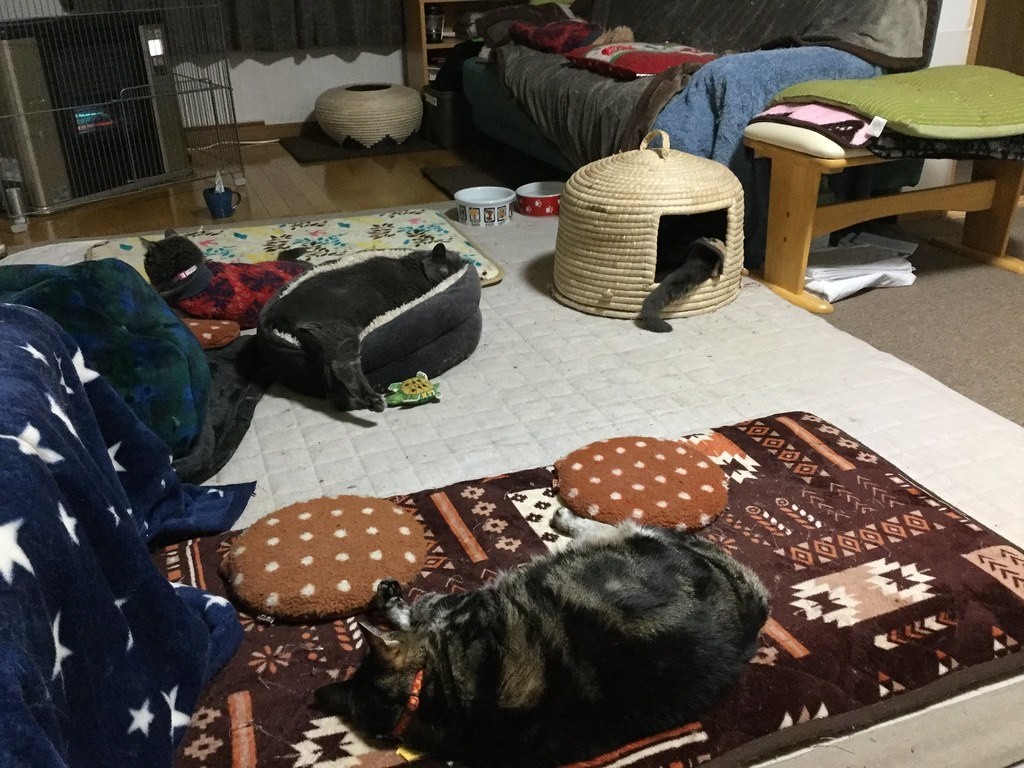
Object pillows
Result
[509,21,605,54]
[563,40,720,81]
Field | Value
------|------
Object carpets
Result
[0,185,1024,766]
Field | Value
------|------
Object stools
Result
[743,66,1023,316]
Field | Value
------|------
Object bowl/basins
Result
[515,181,566,217]
[453,186,517,226]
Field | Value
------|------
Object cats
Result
[634,238,723,333]
[304,507,771,766]
[137,227,315,331]
[261,244,467,411]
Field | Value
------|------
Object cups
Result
[203,187,242,219]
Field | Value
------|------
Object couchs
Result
[423,0,945,226]
